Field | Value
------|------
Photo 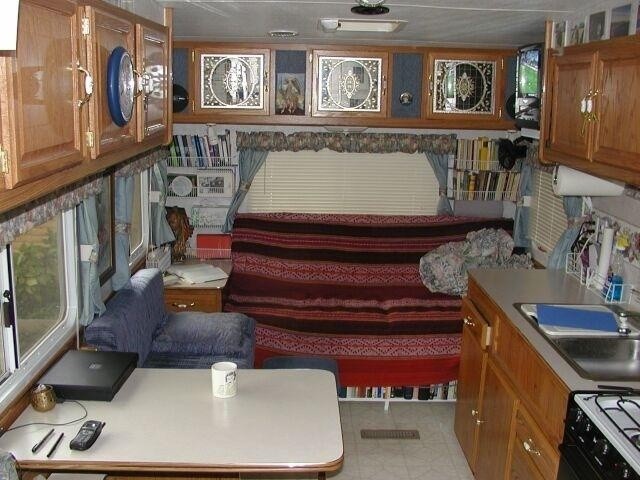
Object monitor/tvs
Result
[514,42,544,131]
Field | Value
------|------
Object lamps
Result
[317,18,408,33]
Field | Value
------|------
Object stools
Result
[260,356,340,394]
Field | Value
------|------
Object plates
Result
[172,176,192,196]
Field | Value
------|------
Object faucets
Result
[618,310,639,318]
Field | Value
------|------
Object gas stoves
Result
[565,390,640,480]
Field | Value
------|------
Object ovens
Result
[557,428,609,480]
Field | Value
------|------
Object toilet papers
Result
[595,227,618,290]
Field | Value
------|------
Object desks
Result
[0,367,346,480]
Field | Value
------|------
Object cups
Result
[212,362,238,398]
[618,313,631,334]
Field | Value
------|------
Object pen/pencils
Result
[32,429,54,452]
[46,432,64,458]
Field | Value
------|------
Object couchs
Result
[83,264,257,369]
[221,212,527,413]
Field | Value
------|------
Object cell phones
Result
[69,420,105,451]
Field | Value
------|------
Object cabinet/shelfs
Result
[162,259,232,312]
[451,135,522,200]
[453,273,573,479]
[172,35,519,129]
[539,2,638,188]
[166,130,236,200]
[0,0,173,212]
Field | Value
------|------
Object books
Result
[449,138,522,200]
[169,129,232,166]
[167,263,228,283]
[340,380,457,400]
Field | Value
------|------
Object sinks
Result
[555,334,640,381]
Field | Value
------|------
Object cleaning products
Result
[602,266,623,300]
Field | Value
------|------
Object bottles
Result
[604,274,622,300]
